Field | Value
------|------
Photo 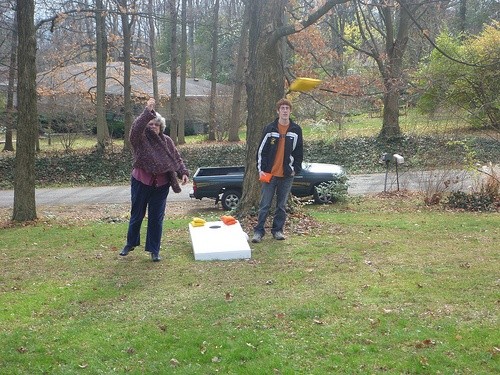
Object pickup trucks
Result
[188,155,348,211]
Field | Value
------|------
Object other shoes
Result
[119,245,135,256]
[151,253,161,261]
[251,230,264,242]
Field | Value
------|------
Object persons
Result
[120,98,190,261]
[251,98,303,243]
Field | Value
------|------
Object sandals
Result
[273,231,285,240]
